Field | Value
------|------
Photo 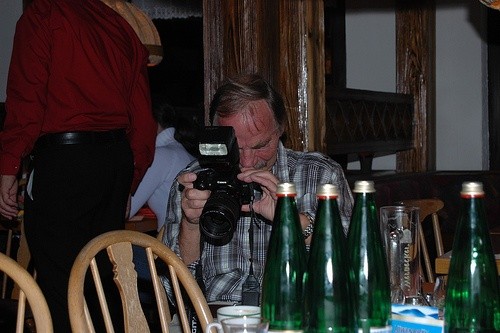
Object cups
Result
[205,305,262,333]
[379,203,423,305]
[221,318,270,333]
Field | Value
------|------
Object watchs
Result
[300,212,316,239]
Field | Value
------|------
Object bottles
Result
[303,183,354,333]
[344,181,393,333]
[443,181,500,333]
[261,182,308,332]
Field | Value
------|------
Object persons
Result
[152,73,355,333]
[0,0,195,333]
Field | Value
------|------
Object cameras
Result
[193,126,263,248]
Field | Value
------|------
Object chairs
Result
[403,199,445,295]
[67,229,217,333]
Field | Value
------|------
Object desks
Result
[436,226,500,290]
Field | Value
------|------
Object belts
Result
[37,128,126,145]
